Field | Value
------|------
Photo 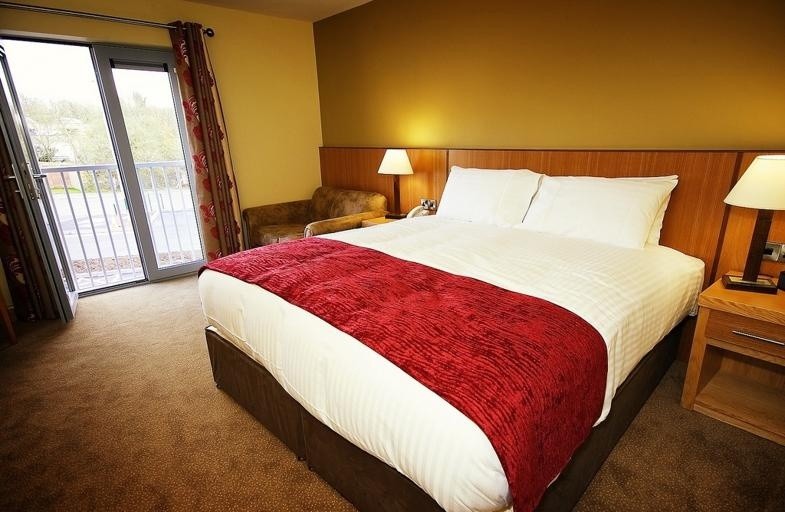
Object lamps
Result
[722,155,785,294]
[378,149,414,220]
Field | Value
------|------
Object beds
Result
[198,215,705,512]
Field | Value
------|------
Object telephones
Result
[406,206,430,218]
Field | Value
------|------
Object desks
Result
[680,270,785,446]
[362,217,396,227]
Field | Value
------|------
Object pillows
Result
[435,166,678,249]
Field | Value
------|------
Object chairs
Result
[241,187,388,249]
